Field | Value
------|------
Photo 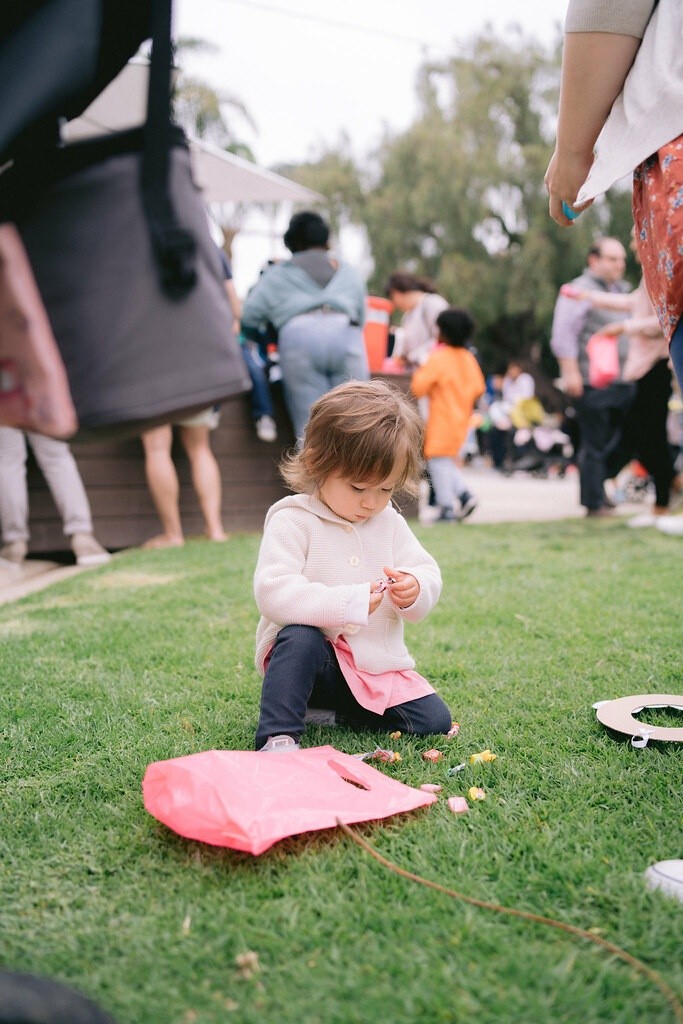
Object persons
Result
[470,354,582,478]
[385,272,452,521]
[238,211,370,456]
[251,376,452,753]
[411,307,487,526]
[138,405,226,550]
[544,0,683,535]
[0,424,111,565]
[548,232,634,522]
[559,222,673,528]
[240,259,280,443]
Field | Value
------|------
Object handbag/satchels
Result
[586,333,621,387]
[141,744,440,856]
[0,121,255,440]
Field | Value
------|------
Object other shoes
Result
[258,735,298,751]
[436,506,457,521]
[302,708,336,726]
[256,415,277,442]
[458,492,476,518]
[209,411,221,431]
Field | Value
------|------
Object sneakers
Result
[645,859,683,902]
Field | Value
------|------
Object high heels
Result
[71,534,111,565]
[0,543,27,570]
